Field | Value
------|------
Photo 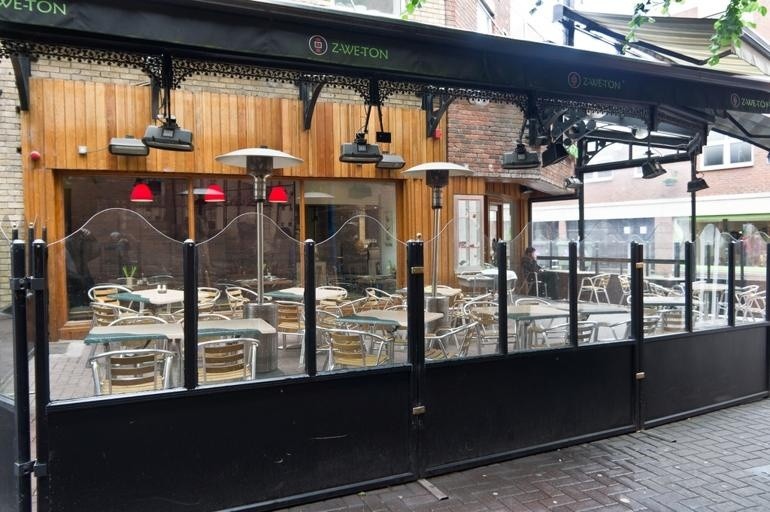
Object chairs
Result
[176,313,234,366]
[317,325,397,372]
[88,348,176,396]
[85,270,461,354]
[182,337,261,381]
[457,270,768,356]
[105,313,171,380]
[420,321,479,360]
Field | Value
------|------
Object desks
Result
[339,307,444,363]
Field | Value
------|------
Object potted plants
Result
[122,264,135,289]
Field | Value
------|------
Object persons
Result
[65,226,102,308]
[520,246,560,300]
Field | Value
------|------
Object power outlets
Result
[79,146,86,153]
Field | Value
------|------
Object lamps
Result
[377,153,406,169]
[212,144,304,372]
[502,114,541,170]
[109,133,150,157]
[401,160,474,323]
[542,130,567,166]
[566,175,583,189]
[684,153,708,191]
[141,56,194,152]
[131,181,287,204]
[642,132,666,179]
[338,94,382,163]
